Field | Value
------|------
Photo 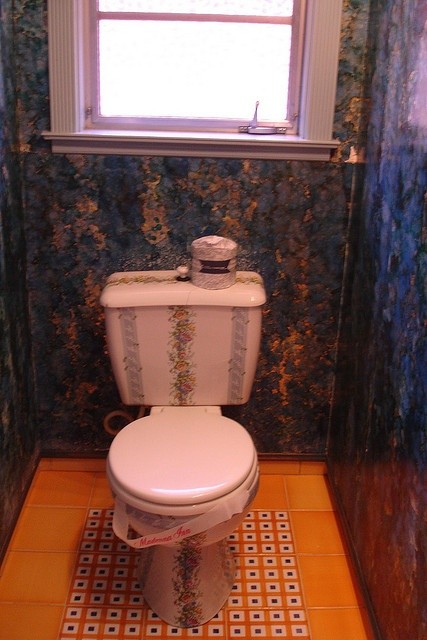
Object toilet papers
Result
[190,236,238,290]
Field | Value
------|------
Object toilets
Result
[98,268,267,630]
